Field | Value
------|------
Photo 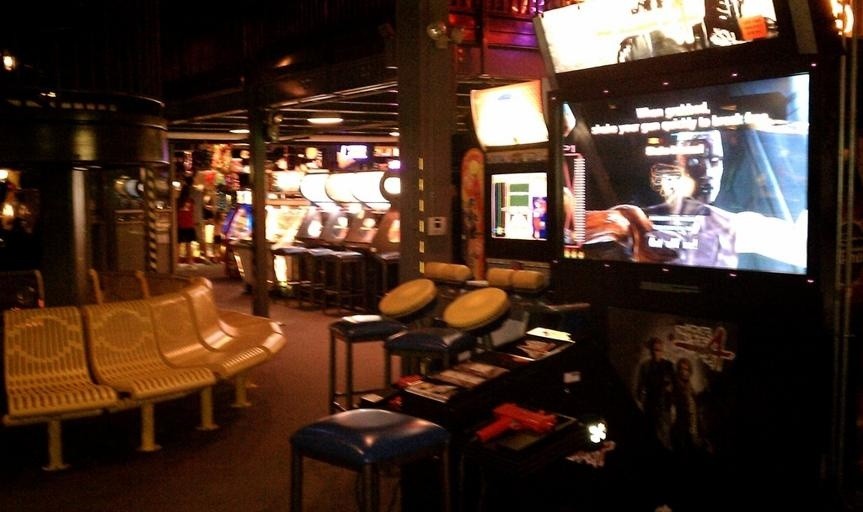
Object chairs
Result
[2,269,285,471]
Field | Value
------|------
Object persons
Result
[177,186,197,264]
[206,207,227,257]
[563,102,808,273]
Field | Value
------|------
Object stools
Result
[289,408,453,511]
[386,327,475,385]
[273,246,367,315]
[373,250,401,296]
[328,314,404,418]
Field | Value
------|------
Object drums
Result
[377,278,438,322]
[444,288,512,337]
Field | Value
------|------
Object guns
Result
[476,403,556,444]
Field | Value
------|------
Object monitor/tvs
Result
[482,163,549,261]
[549,33,819,325]
